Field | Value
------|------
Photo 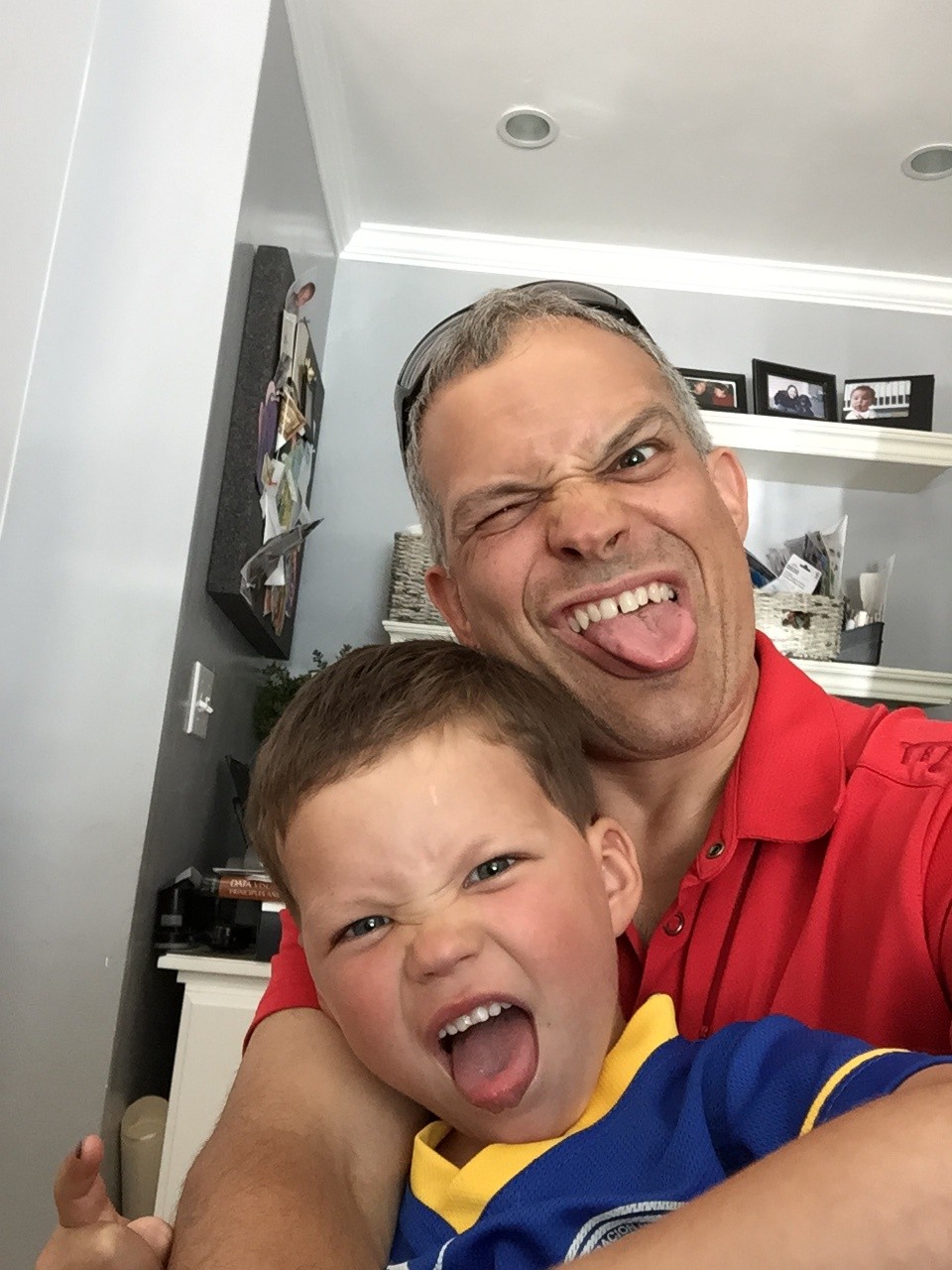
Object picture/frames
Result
[752,359,838,421]
[677,368,748,413]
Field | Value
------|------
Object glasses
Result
[394,279,654,469]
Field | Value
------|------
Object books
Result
[218,875,285,902]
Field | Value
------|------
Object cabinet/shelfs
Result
[378,410,952,708]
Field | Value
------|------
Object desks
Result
[151,953,270,1226]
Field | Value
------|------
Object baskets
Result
[750,590,847,660]
[388,535,454,626]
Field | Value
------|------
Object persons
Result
[38,638,952,1270]
[164,280,952,1270]
[286,282,315,315]
[774,385,802,413]
[845,385,879,420]
[690,381,733,407]
